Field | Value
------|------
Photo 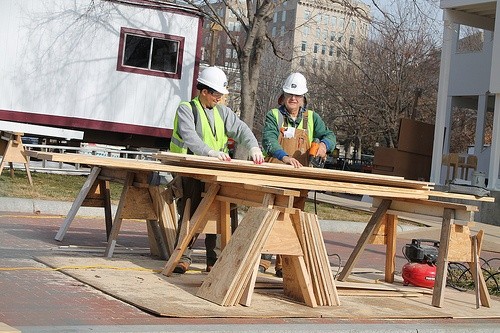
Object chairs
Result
[445,152,479,187]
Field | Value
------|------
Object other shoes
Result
[173,249,192,273]
[275,255,282,277]
[258,253,271,273]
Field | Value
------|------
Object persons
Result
[166,66,265,273]
[258,72,337,277]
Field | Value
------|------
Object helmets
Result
[282,72,308,95]
[197,67,230,95]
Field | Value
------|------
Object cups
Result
[470,172,486,188]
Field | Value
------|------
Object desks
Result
[23,150,496,311]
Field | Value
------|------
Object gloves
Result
[208,149,231,162]
[248,147,264,164]
